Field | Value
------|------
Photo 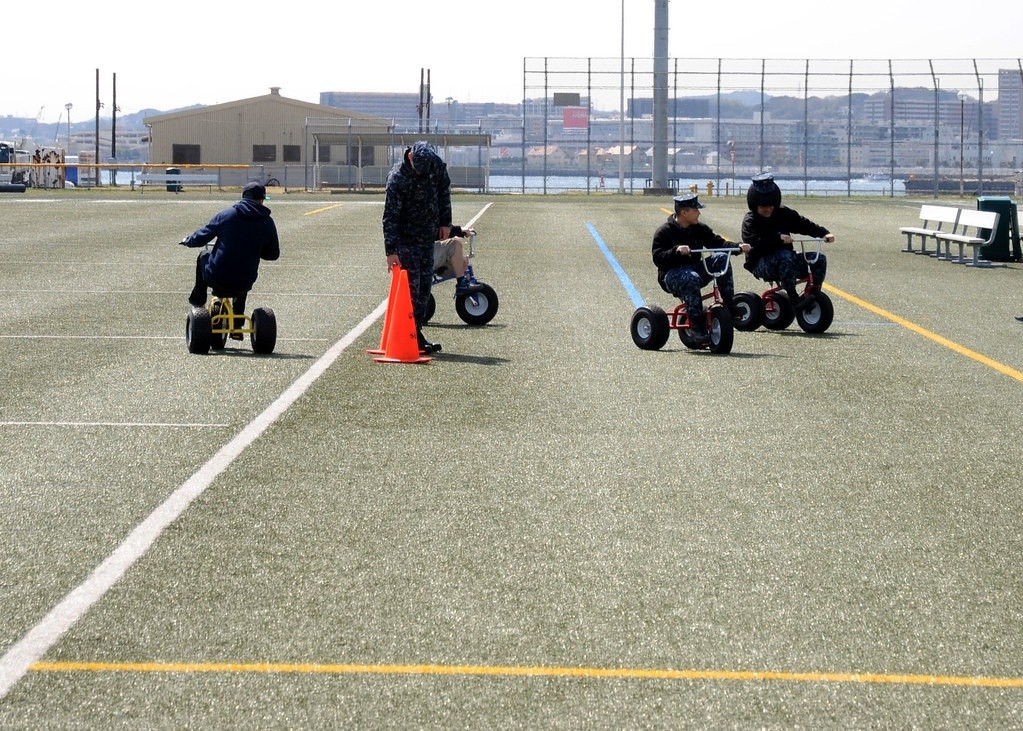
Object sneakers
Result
[455,282,484,296]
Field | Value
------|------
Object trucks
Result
[0,140,31,192]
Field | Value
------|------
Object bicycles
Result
[248,173,280,187]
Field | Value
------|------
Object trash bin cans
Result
[166,168,183,192]
[976,195,1011,261]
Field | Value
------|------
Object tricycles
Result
[418,227,500,326]
[630,244,751,355]
[176,237,277,355]
[731,236,836,335]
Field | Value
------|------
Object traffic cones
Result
[364,263,426,355]
[372,269,433,364]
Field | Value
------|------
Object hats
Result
[242,183,270,201]
[412,142,435,175]
[673,192,704,208]
[752,171,775,193]
[747,182,781,213]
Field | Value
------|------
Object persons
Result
[182,181,280,341]
[433,224,483,296]
[741,172,834,310]
[382,141,452,354]
[652,194,751,343]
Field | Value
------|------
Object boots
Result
[724,296,746,318]
[787,287,815,312]
[417,328,441,353]
[692,316,710,343]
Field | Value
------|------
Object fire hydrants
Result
[706,180,714,196]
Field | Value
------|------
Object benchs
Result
[935,208,1009,270]
[131,171,219,195]
[898,203,961,261]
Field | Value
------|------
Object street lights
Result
[65,103,73,155]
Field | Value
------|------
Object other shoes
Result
[232,327,243,339]
